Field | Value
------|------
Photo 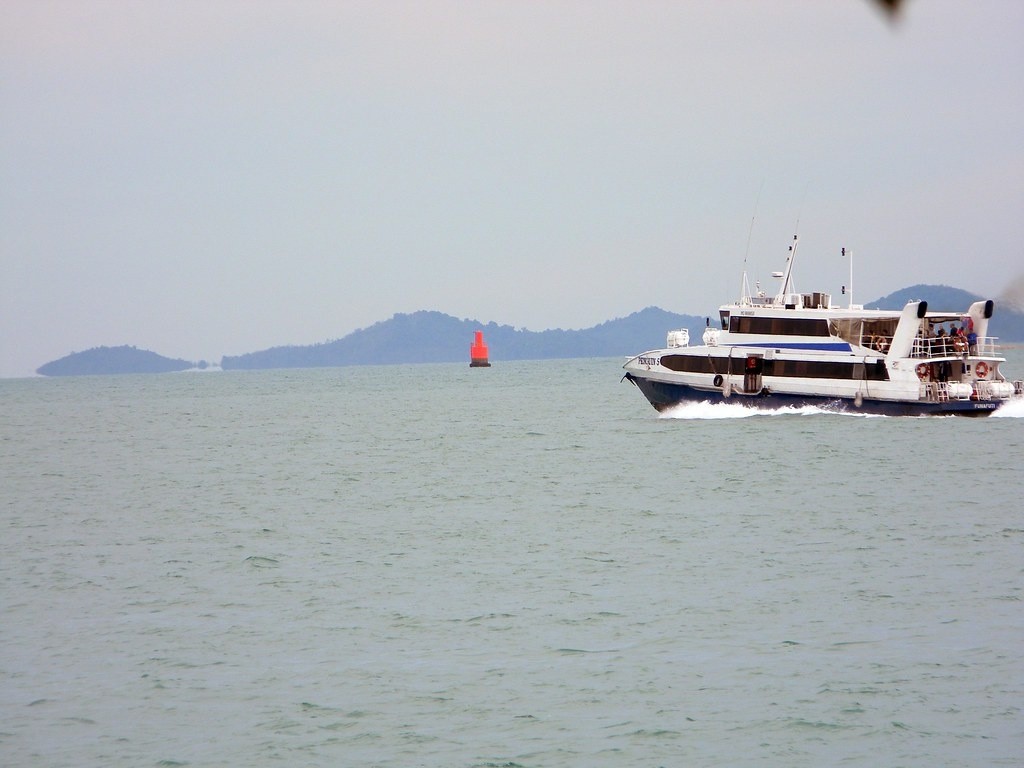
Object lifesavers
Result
[952,335,967,352]
[917,363,930,379]
[713,375,724,387]
[876,337,887,351]
[975,362,989,378]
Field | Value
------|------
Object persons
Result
[866,322,978,357]
[938,362,948,391]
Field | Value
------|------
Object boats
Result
[619,232,1016,419]
